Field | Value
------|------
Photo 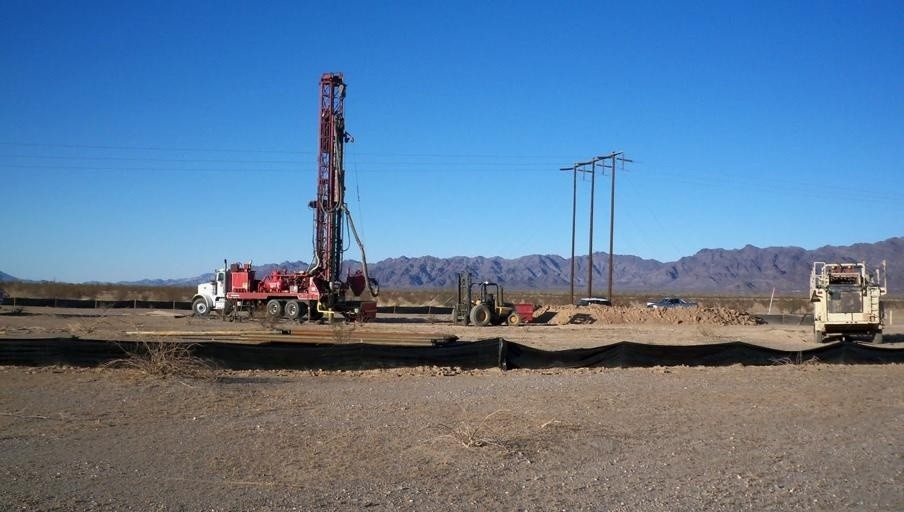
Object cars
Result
[647,298,699,310]
[576,297,613,309]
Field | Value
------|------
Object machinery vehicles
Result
[451,282,533,326]
[808,260,888,345]
[189,71,380,324]
